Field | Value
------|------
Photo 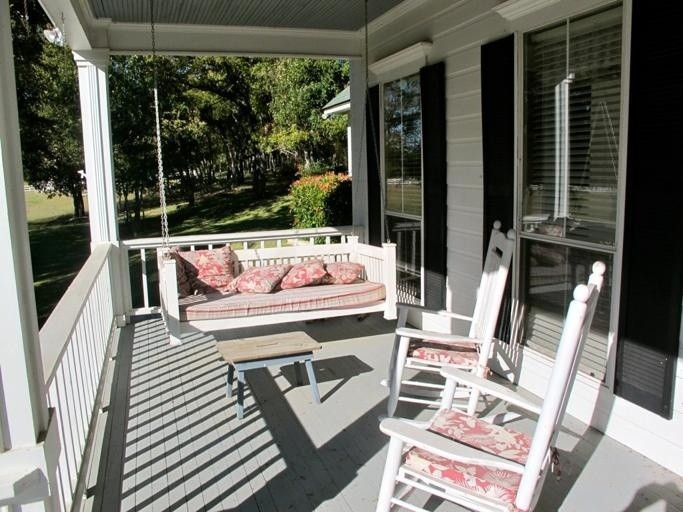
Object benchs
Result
[157,236,396,346]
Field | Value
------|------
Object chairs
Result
[377,221,515,421]
[375,260,607,512]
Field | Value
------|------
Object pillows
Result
[225,263,291,293]
[322,263,364,284]
[163,246,190,297]
[277,259,327,289]
[179,244,235,295]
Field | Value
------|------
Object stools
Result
[215,332,321,420]
